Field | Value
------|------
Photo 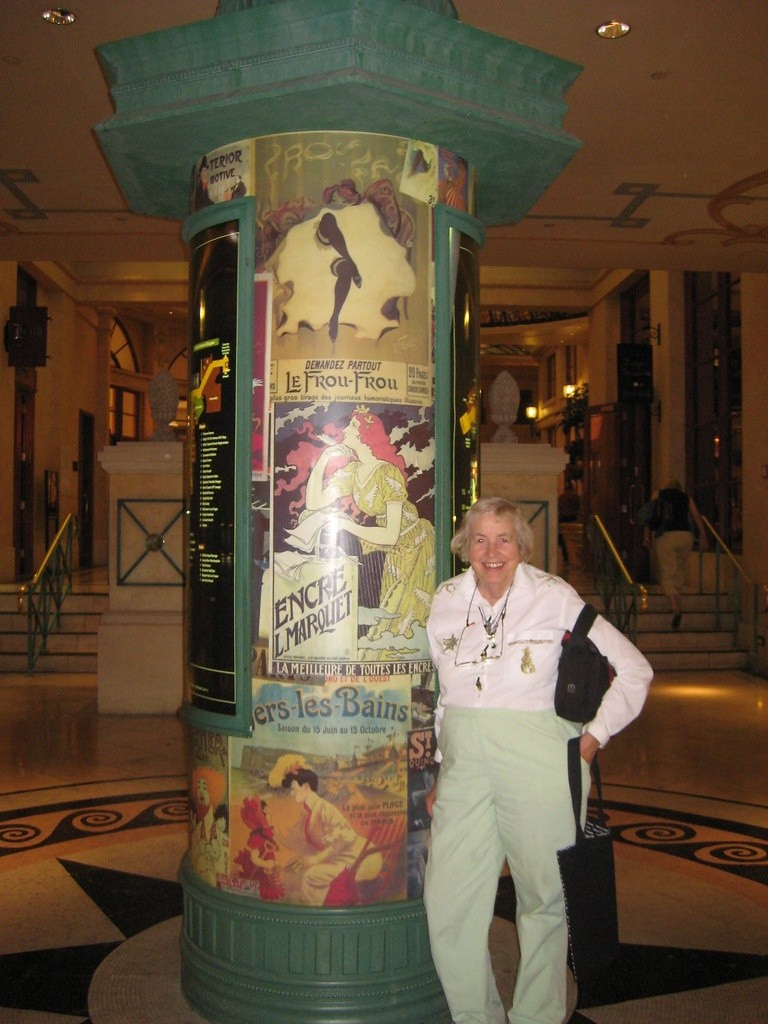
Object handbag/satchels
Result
[554,603,612,722]
[557,739,624,1008]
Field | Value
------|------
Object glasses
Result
[454,613,507,670]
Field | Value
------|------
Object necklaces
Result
[475,583,513,656]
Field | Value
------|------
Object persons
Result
[643,475,709,628]
[422,497,653,1024]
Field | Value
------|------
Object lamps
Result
[562,383,575,399]
[525,402,537,419]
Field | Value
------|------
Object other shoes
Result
[671,612,685,629]
